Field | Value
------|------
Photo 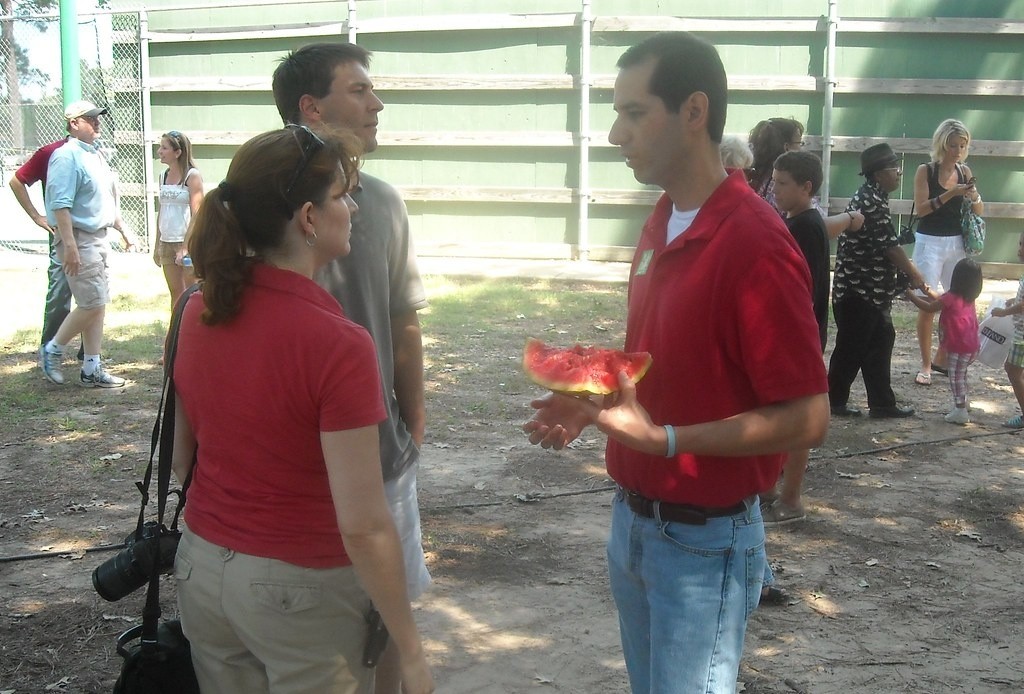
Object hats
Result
[857,143,904,177]
[64,100,108,120]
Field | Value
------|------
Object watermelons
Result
[522,337,654,396]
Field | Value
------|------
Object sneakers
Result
[41,341,65,385]
[79,365,126,387]
[944,407,969,423]
[76,350,111,364]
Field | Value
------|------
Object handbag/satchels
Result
[110,618,202,694]
[975,313,1015,370]
[954,163,986,257]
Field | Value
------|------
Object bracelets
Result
[663,425,676,459]
[847,210,854,229]
[923,285,930,294]
[935,195,943,206]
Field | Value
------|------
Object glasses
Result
[788,140,806,146]
[283,124,325,220]
[882,166,902,175]
[169,130,184,148]
[76,115,100,123]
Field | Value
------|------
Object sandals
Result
[759,586,790,600]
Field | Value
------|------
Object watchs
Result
[970,193,981,204]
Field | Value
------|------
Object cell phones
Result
[967,176,977,186]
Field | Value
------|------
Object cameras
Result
[896,228,915,245]
[92,522,184,602]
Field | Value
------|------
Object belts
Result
[625,490,757,526]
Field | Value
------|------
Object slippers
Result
[914,371,932,386]
[1002,417,1024,428]
[932,362,949,376]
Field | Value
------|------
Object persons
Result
[8,135,88,366]
[519,31,827,694]
[38,96,136,389]
[903,258,983,423]
[827,145,926,420]
[273,41,433,694]
[743,117,866,475]
[989,231,1024,430]
[758,150,829,528]
[720,132,754,170]
[160,122,433,694]
[153,131,205,366]
[913,118,984,384]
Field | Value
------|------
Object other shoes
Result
[829,403,861,416]
[869,402,915,418]
[761,502,806,527]
